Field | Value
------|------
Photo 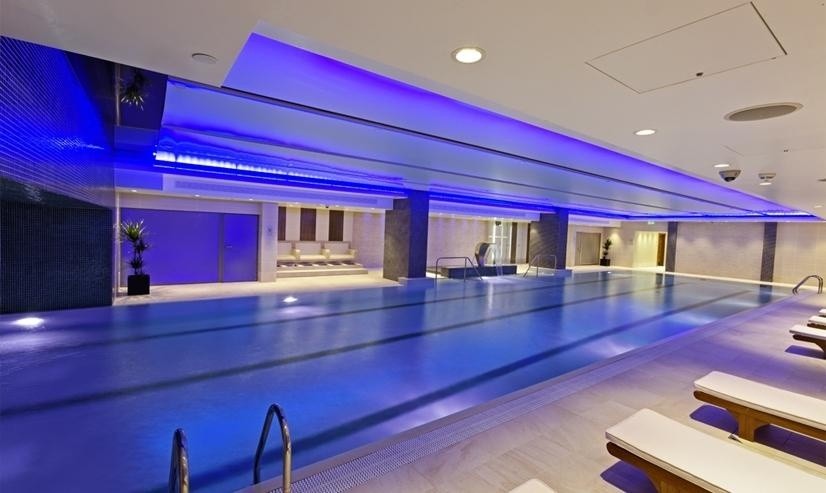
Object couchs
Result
[277,240,357,263]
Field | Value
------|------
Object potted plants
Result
[600,239,612,266]
[114,218,152,295]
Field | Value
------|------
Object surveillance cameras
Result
[718,170,741,181]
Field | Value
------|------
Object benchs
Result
[694,370,825,442]
[807,316,825,328]
[512,479,553,491]
[605,408,825,491]
[818,310,825,317]
[789,325,825,351]
